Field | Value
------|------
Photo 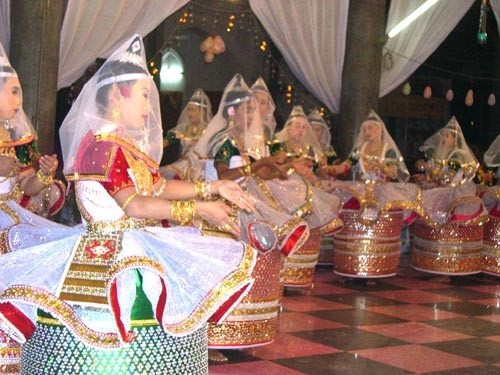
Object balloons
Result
[488,93,495,105]
[200,35,226,63]
[401,81,412,97]
[463,89,475,107]
[424,86,433,100]
[445,88,454,102]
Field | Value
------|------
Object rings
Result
[6,167,17,178]
[216,217,236,233]
[49,161,58,171]
[239,195,244,202]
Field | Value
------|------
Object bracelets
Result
[36,169,53,185]
[171,197,196,224]
[195,179,212,198]
[238,164,251,177]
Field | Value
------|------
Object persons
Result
[410,116,500,278]
[316,109,422,284]
[0,40,66,219]
[162,74,340,361]
[1,34,67,375]
[0,35,259,375]
